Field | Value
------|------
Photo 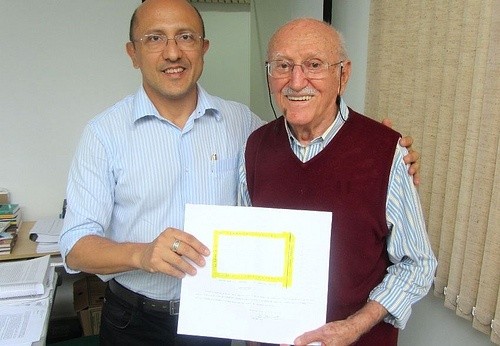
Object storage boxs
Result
[73,278,106,336]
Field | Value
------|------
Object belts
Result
[109,278,180,315]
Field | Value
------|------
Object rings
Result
[172,239,180,252]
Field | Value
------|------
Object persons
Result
[239,18,438,346]
[59,0,421,346]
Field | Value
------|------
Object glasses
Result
[266,57,344,78]
[132,32,205,49]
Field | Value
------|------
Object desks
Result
[0,272,58,346]
[0,221,64,261]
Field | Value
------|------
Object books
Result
[0,188,23,254]
